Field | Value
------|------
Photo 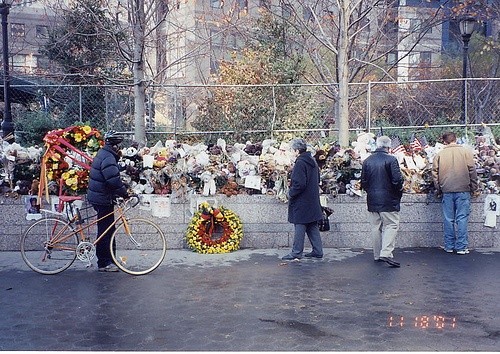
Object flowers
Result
[184,202,244,255]
[36,120,104,205]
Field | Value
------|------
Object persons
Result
[361,136,404,267]
[431,131,478,254]
[281,138,324,260]
[87,130,130,272]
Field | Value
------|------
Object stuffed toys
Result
[235,160,255,178]
[486,181,499,194]
[197,171,217,196]
[137,140,177,168]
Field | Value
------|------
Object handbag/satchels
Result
[318,206,335,231]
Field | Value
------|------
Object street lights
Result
[0,0,15,147]
[457,10,476,126]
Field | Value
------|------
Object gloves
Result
[128,192,138,197]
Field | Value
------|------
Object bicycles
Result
[20,193,166,277]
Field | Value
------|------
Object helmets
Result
[104,129,124,146]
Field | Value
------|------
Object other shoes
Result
[282,253,302,260]
[374,257,400,267]
[444,248,453,253]
[99,263,119,272]
[457,250,470,255]
[305,251,322,258]
